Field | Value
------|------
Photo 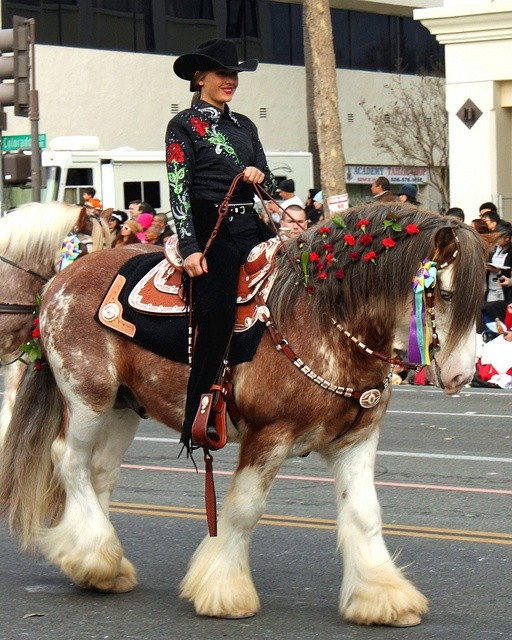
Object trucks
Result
[12,138,316,237]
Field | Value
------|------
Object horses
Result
[0,202,115,369]
[0,200,489,627]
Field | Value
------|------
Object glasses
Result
[108,218,115,221]
[121,225,130,231]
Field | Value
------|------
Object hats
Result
[125,221,139,233]
[174,39,259,81]
[397,184,416,199]
[112,210,127,223]
[136,213,153,229]
[313,191,323,205]
[275,179,294,192]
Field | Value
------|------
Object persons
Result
[135,203,156,228]
[470,220,496,256]
[111,220,142,248]
[445,207,464,224]
[82,188,103,211]
[371,176,396,202]
[108,211,128,236]
[481,211,500,231]
[144,225,163,247]
[128,200,141,220]
[312,190,325,222]
[485,222,512,342]
[304,188,318,220]
[479,202,512,230]
[165,40,277,446]
[280,205,308,238]
[396,183,418,208]
[268,179,306,223]
[470,303,512,390]
[152,213,174,242]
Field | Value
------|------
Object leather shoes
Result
[207,426,219,441]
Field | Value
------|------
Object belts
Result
[223,203,257,213]
